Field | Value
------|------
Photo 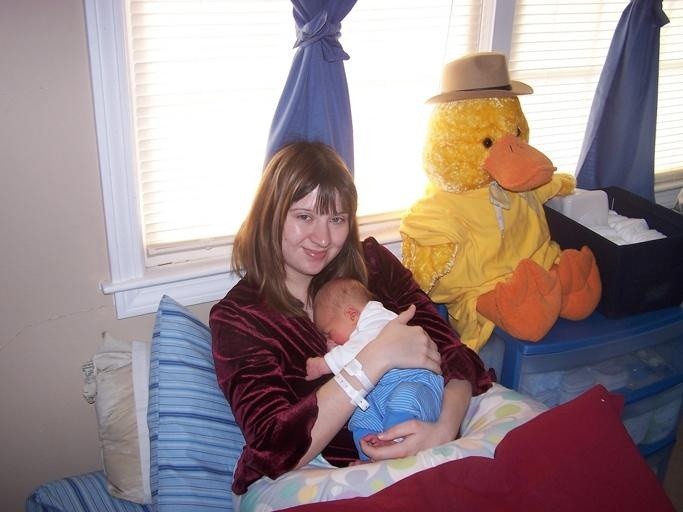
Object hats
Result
[425,51,533,103]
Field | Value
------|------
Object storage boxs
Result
[542,185,683,318]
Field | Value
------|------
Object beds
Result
[30,296,683,512]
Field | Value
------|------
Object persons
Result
[305,278,446,460]
[209,140,495,495]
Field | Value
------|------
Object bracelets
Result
[332,358,374,412]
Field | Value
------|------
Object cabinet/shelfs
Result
[438,302,682,481]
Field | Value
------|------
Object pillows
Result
[148,295,247,512]
[81,334,153,505]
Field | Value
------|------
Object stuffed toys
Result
[399,50,603,352]
